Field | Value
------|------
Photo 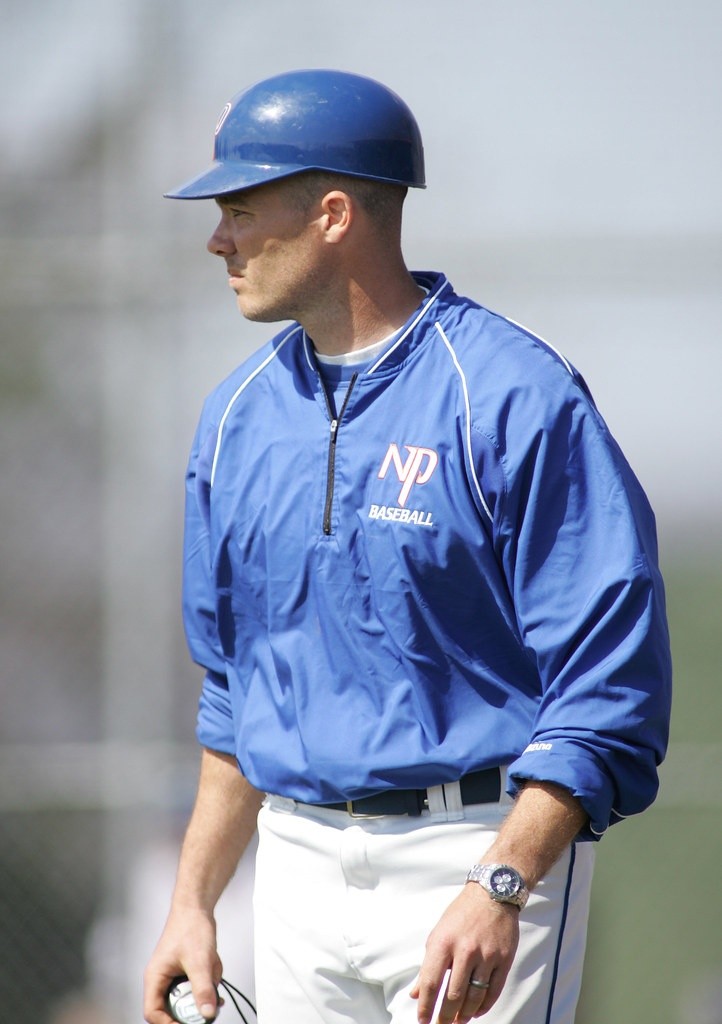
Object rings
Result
[471,979,489,989]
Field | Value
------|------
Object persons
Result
[141,68,674,1024]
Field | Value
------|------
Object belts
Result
[294,766,501,818]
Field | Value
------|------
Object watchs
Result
[466,862,530,912]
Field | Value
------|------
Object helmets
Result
[161,69,428,199]
